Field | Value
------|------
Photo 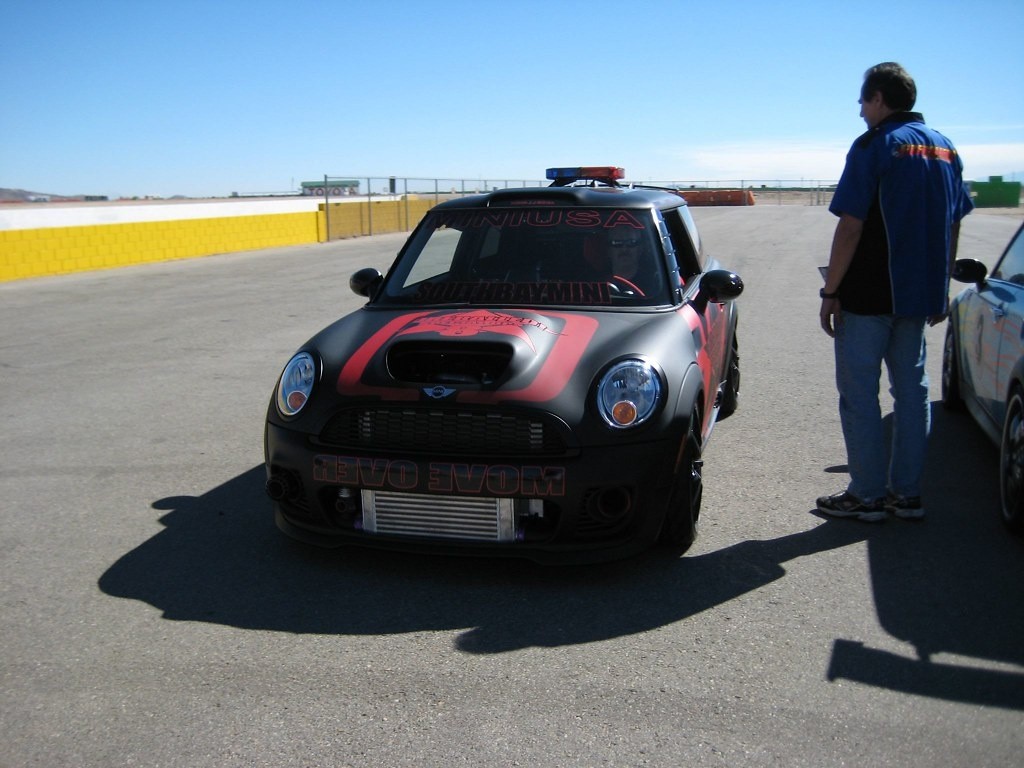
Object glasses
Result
[607,238,639,248]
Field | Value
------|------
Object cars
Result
[263,166,746,564]
[940,216,1024,537]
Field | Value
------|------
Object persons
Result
[816,62,975,521]
[581,224,665,307]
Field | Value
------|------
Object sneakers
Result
[816,491,888,521]
[884,488,924,517]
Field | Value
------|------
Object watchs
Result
[820,288,836,299]
